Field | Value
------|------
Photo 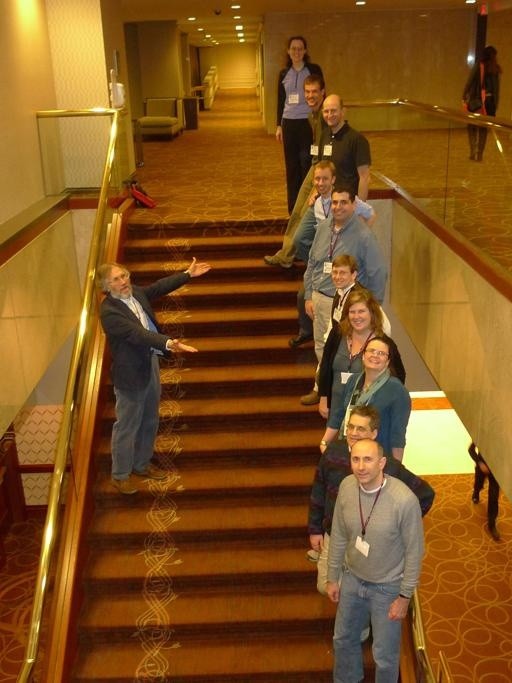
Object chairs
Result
[136,98,187,140]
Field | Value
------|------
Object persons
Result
[307,403,435,647]
[291,93,372,269]
[317,288,407,431]
[300,184,388,406]
[274,34,327,216]
[93,256,213,495]
[324,436,427,683]
[288,159,377,348]
[468,441,500,542]
[322,252,391,348]
[263,72,332,268]
[304,334,412,563]
[460,45,503,163]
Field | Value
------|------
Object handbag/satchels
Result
[468,100,482,112]
[132,180,156,208]
[462,99,487,116]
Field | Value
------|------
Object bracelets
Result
[398,593,411,601]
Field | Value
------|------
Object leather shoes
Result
[472,492,479,504]
[490,526,499,540]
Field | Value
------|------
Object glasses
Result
[364,348,389,357]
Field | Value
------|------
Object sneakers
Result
[111,476,137,495]
[144,465,169,479]
[289,335,309,346]
[263,255,292,269]
[305,549,320,561]
[300,390,319,405]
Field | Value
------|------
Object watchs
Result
[320,440,330,447]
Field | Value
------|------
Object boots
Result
[469,125,478,161]
[478,128,487,160]
